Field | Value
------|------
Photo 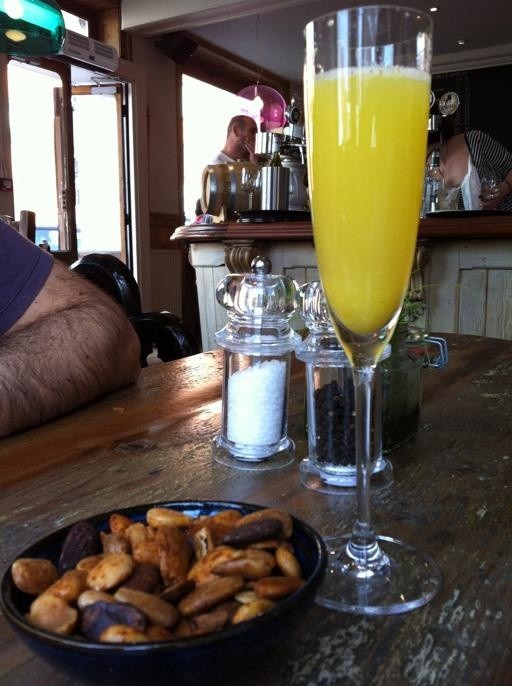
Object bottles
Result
[294,279,398,495]
[429,148,443,210]
[217,259,298,475]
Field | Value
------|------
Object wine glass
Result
[303,7,443,617]
[239,167,260,210]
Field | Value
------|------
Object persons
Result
[427,107,508,212]
[0,207,142,441]
[209,114,250,170]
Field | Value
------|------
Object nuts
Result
[11,506,306,645]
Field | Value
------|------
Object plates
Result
[439,92,460,115]
[234,209,309,223]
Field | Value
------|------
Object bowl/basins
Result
[2,502,328,680]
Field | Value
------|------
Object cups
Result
[260,166,289,211]
[480,176,500,210]
